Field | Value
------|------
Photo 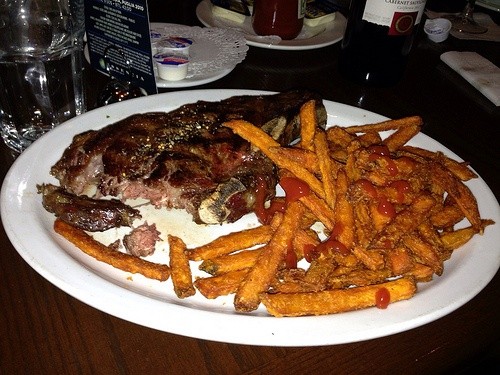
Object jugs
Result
[97,45,148,108]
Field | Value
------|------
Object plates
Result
[84,22,239,88]
[196,0,348,51]
[0,89,500,348]
[466,0,500,12]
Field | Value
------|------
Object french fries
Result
[51,99,495,318]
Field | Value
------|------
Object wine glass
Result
[440,0,488,34]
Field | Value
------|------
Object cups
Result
[151,30,162,56]
[158,36,193,56]
[423,17,452,43]
[0,0,86,159]
[153,52,189,81]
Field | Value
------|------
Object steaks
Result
[42,93,328,260]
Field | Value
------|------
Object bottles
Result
[336,0,427,89]
[252,0,306,40]
